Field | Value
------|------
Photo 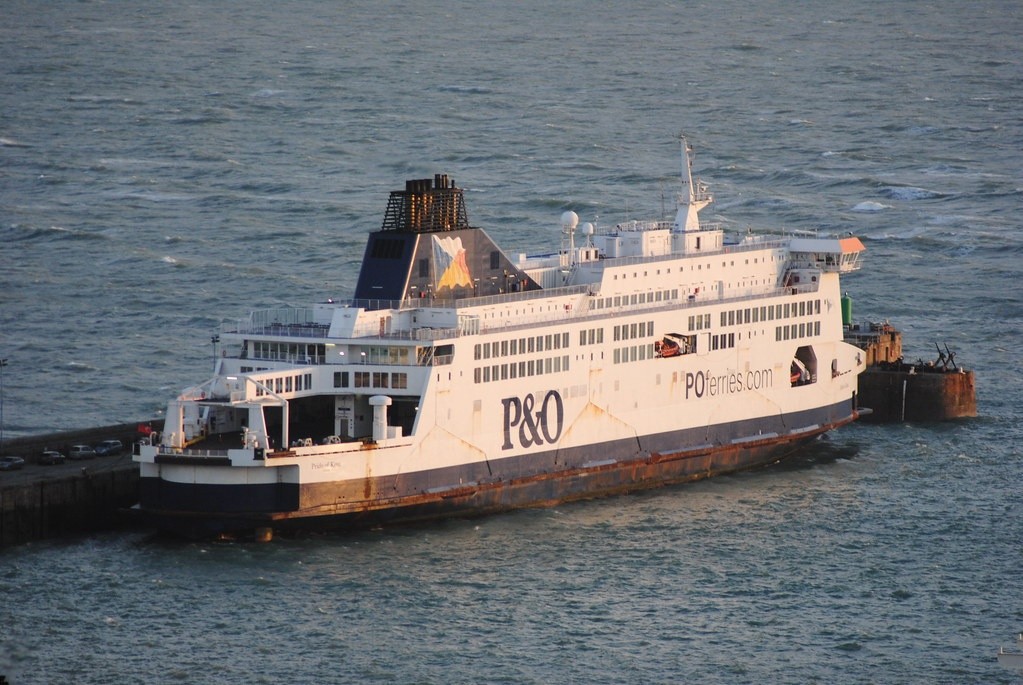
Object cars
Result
[69,445,98,459]
[0,456,25,471]
[42,451,66,465]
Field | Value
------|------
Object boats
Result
[791,370,801,383]
[130,131,876,544]
[654,337,684,357]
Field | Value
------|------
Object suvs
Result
[95,440,123,457]
